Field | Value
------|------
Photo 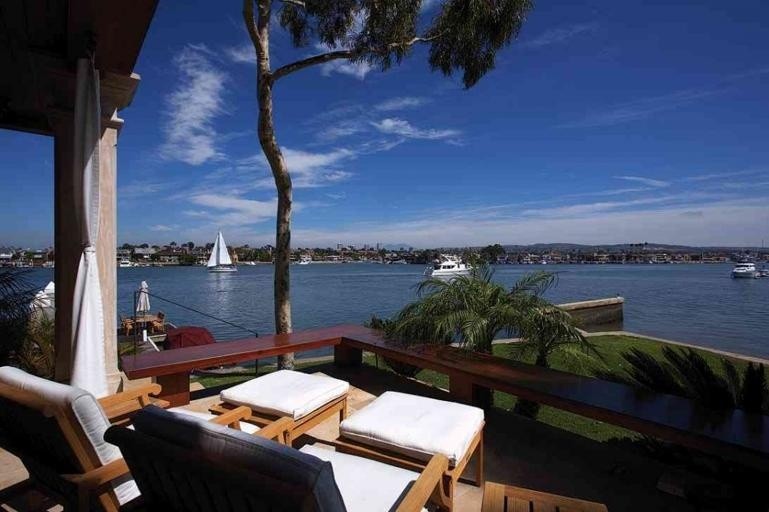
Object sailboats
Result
[207,231,238,272]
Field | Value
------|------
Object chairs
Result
[2,367,295,510]
[103,405,455,511]
[117,312,164,337]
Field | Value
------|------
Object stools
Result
[220,368,347,449]
[334,389,485,486]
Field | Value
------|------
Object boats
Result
[422,261,472,276]
[731,262,769,278]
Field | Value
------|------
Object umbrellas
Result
[136,279,151,318]
